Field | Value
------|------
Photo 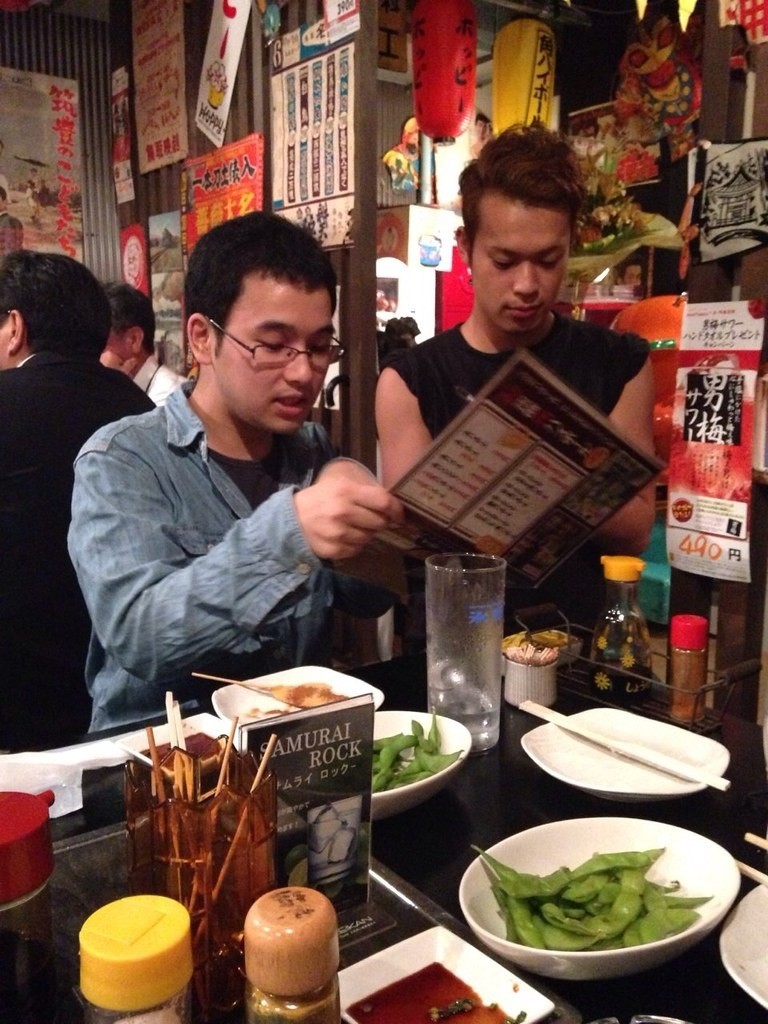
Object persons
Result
[0,248,158,753]
[66,211,404,734]
[375,123,659,657]
[89,281,189,406]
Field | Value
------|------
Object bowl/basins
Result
[457,817,740,981]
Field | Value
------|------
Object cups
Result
[502,658,557,710]
[424,549,506,754]
[123,732,273,1024]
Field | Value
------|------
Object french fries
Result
[500,628,570,649]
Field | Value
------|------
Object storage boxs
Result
[435,246,475,335]
[0,650,767,1024]
[374,204,458,272]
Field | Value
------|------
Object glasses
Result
[204,315,344,368]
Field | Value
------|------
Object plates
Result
[117,712,245,785]
[495,630,583,677]
[367,710,472,821]
[719,879,768,1013]
[335,925,556,1024]
[520,707,730,801]
[210,664,386,734]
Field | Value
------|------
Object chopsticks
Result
[733,831,768,891]
[519,701,732,792]
[144,692,277,958]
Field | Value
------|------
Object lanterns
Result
[492,19,556,141]
[411,0,476,146]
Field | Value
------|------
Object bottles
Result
[244,885,342,1024]
[0,789,61,1024]
[590,556,654,700]
[670,614,709,722]
[77,894,192,1024]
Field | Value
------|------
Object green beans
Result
[373,709,466,792]
[467,842,710,951]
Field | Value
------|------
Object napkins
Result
[0,741,128,794]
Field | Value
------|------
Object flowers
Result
[565,128,685,281]
